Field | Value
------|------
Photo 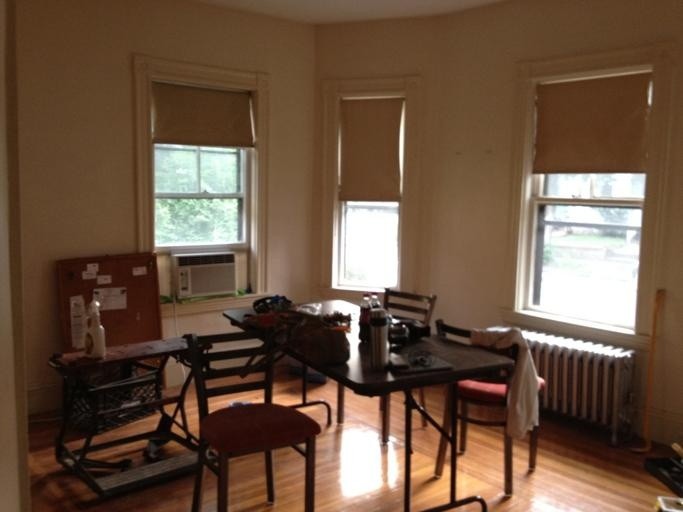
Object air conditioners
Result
[172,252,237,300]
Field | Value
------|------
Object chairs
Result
[186,328,320,511]
[336,287,437,446]
[434,316,546,496]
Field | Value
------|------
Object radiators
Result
[486,326,635,445]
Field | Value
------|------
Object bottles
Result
[359,293,372,342]
[371,308,388,368]
[372,292,381,308]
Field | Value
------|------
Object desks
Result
[222,295,516,494]
[51,335,207,499]
